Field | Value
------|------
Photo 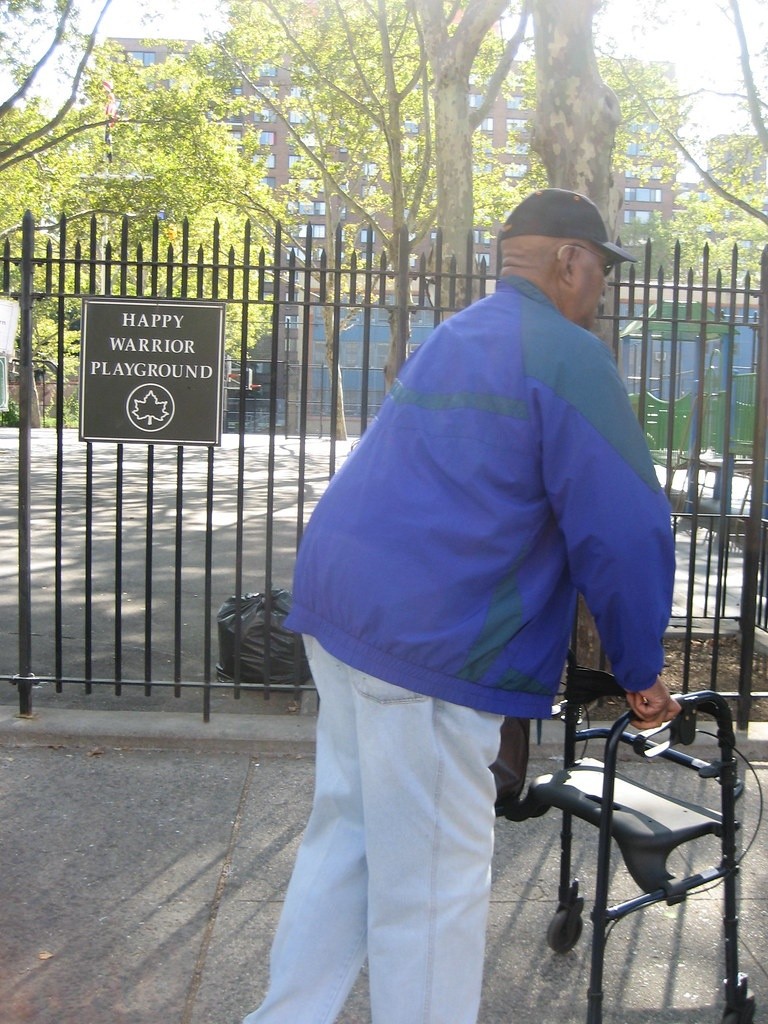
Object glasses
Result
[560,242,614,278]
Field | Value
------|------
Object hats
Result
[497,189,637,268]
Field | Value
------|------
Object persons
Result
[232,184,683,1023]
